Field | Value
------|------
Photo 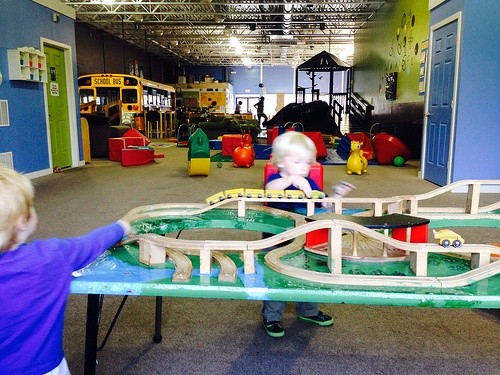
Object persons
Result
[254,96,268,130]
[261,131,373,337]
[234,101,242,114]
[0,165,132,375]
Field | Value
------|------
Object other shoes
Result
[298,309,333,326]
[262,319,285,338]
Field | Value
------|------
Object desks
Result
[70,208,500,375]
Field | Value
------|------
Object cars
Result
[432,229,465,248]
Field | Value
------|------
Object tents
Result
[296,50,353,104]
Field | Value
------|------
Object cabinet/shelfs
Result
[7,48,48,84]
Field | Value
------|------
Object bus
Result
[78,74,176,132]
[162,76,235,115]
